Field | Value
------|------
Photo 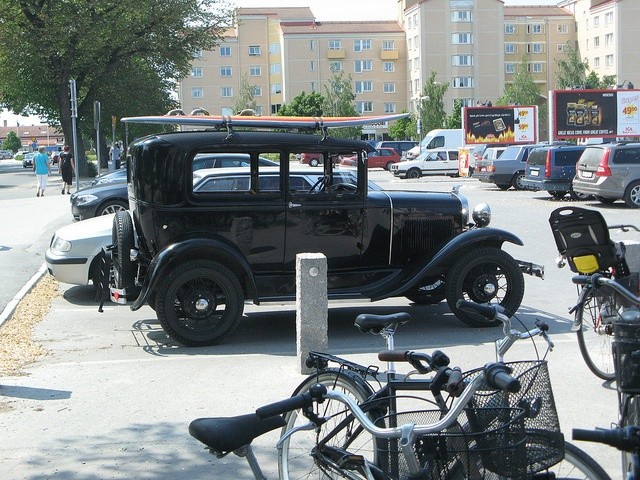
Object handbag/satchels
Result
[33,164,36,173]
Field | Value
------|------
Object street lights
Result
[411,96,429,153]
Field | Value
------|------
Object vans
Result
[400,129,485,161]
[0,149,13,160]
[374,141,418,158]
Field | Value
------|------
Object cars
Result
[13,151,28,159]
[46,166,384,302]
[297,153,343,166]
[391,148,476,178]
[476,145,558,190]
[481,148,507,161]
[23,153,35,167]
[50,151,61,165]
[342,148,400,171]
[70,155,279,220]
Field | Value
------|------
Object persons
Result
[60,146,76,195]
[32,146,52,197]
[114,144,121,169]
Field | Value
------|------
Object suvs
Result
[520,146,594,200]
[572,141,640,208]
[106,130,544,346]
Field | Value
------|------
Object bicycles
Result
[257,361,521,480]
[549,207,640,380]
[280,300,612,480]
[573,274,640,480]
[189,349,482,480]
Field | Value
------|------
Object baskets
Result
[373,409,527,479]
[613,322,640,393]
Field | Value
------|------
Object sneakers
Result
[62,189,64,194]
[37,193,39,197]
[41,194,44,196]
[67,191,71,194]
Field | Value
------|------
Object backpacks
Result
[60,157,71,172]
